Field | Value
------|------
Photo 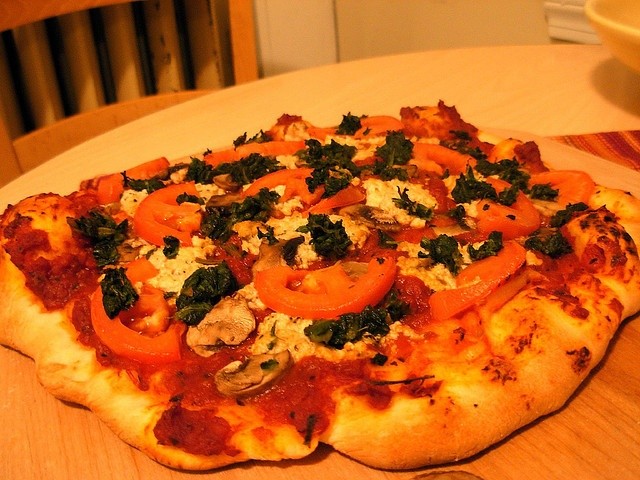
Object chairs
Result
[0,0,258,187]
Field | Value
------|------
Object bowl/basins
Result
[584,0,640,72]
